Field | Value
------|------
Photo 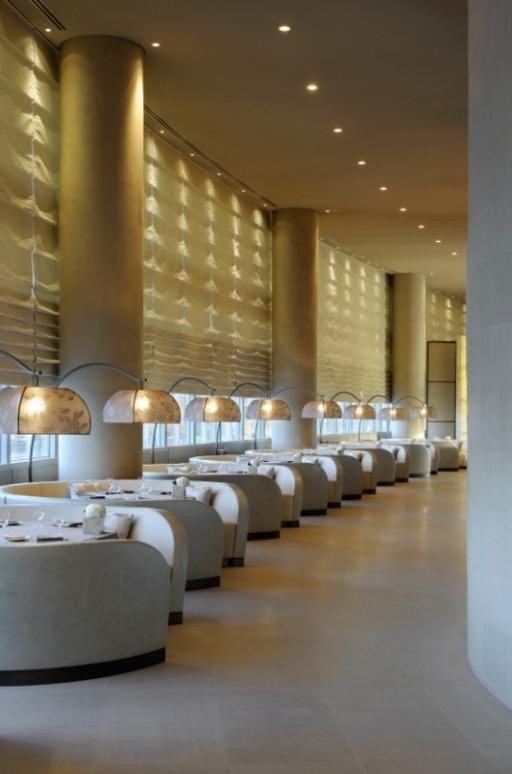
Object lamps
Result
[320,390,377,443]
[0,347,91,437]
[255,384,343,448]
[216,382,292,452]
[24,362,182,483]
[151,376,241,467]
[357,393,408,442]
[387,396,437,439]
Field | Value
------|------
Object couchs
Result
[1,501,187,684]
[297,446,377,502]
[433,440,467,471]
[406,442,439,477]
[192,452,344,518]
[349,443,409,486]
[141,459,302,540]
[0,479,248,589]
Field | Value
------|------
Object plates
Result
[4,535,29,541]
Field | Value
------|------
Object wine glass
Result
[0,511,9,531]
[53,516,64,537]
[33,510,44,528]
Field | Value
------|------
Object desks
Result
[0,519,115,545]
[83,489,182,500]
